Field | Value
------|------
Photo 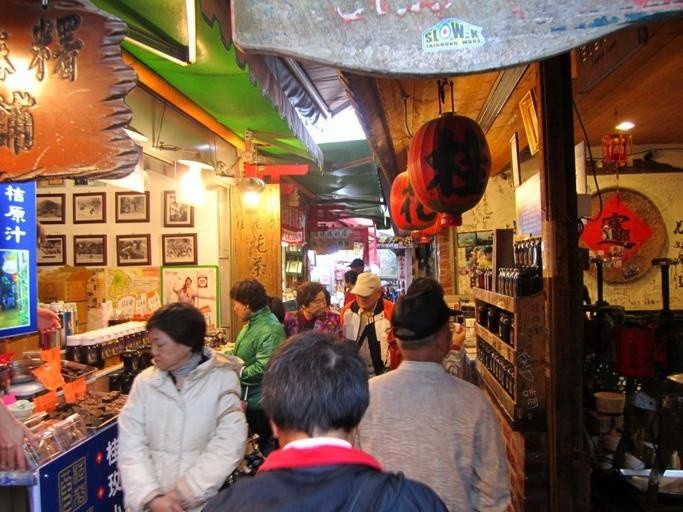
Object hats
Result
[391,293,463,341]
[346,258,364,268]
[349,271,381,298]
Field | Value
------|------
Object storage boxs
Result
[77,303,87,332]
[37,280,88,302]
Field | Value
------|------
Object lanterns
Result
[390,81,492,244]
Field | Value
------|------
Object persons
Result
[216,277,288,456]
[173,273,215,304]
[281,259,474,378]
[470,247,492,268]
[258,294,289,340]
[353,291,511,512]
[194,331,447,512]
[117,302,249,512]
[0,308,62,471]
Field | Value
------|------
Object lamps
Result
[125,124,148,142]
[157,141,215,170]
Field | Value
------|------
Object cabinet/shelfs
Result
[473,286,546,433]
[0,362,130,512]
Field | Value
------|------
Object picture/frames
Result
[164,191,194,226]
[456,232,478,247]
[73,191,107,224]
[37,193,67,223]
[73,234,107,267]
[162,234,197,265]
[116,234,151,267]
[37,235,66,266]
[477,231,493,245]
[116,191,151,223]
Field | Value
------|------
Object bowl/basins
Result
[592,391,626,415]
